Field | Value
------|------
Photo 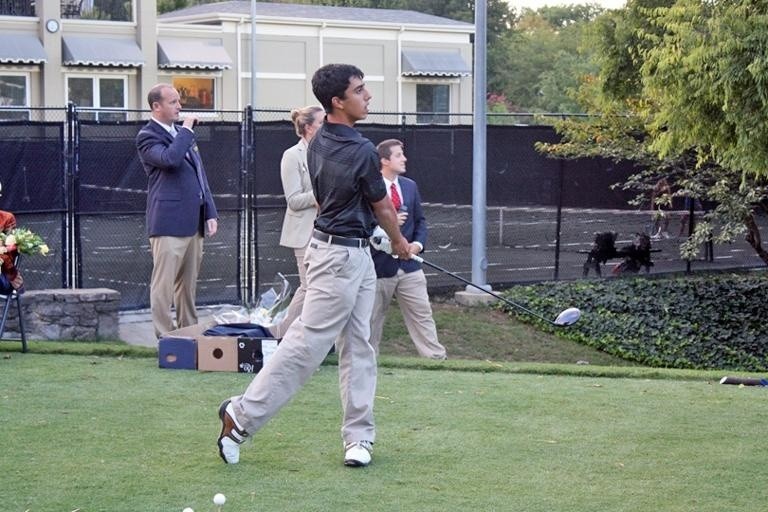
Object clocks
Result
[46,19,59,32]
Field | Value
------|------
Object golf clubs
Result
[373,236,582,328]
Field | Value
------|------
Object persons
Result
[134,82,218,342]
[648,174,673,241]
[217,62,416,469]
[673,177,705,240]
[367,137,449,361]
[1,180,24,300]
[274,104,328,343]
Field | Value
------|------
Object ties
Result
[388,183,402,212]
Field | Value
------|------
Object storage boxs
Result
[158,320,283,373]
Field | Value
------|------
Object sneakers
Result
[340,440,372,466]
[214,395,247,466]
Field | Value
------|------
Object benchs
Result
[577,248,664,277]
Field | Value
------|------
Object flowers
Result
[0,226,49,267]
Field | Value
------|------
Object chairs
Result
[0,252,29,353]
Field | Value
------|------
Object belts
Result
[311,228,371,249]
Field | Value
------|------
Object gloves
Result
[371,224,394,254]
